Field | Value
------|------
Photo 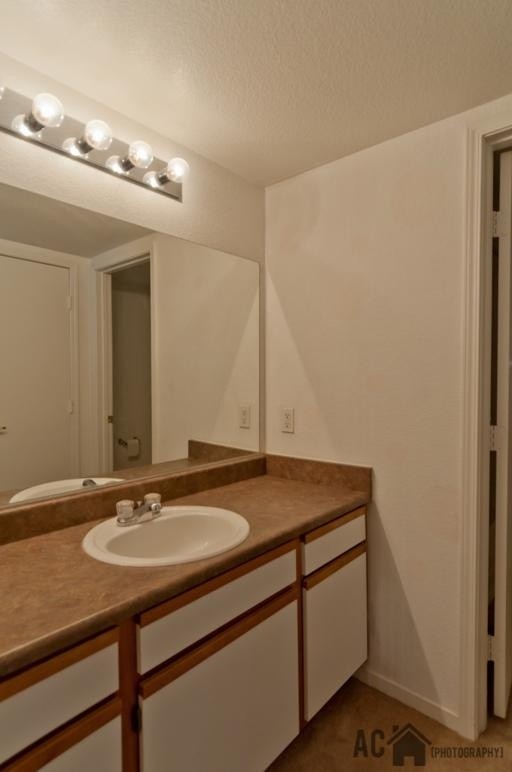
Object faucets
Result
[82,478,94,485]
[143,491,164,515]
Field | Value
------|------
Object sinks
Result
[82,507,250,570]
[9,477,125,506]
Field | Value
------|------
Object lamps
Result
[0,86,190,199]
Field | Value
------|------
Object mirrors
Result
[0,182,262,514]
